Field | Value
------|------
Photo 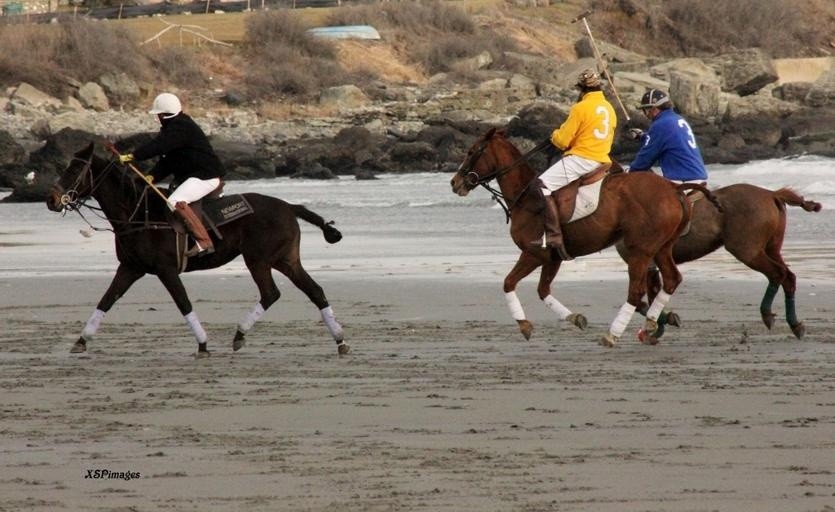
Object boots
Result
[530,195,563,247]
[175,201,215,257]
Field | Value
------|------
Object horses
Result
[448,125,723,349]
[45,139,353,358]
[608,155,822,340]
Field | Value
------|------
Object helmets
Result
[636,88,670,109]
[149,93,181,115]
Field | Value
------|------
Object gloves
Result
[145,175,154,185]
[629,128,643,139]
[624,167,630,173]
[119,154,133,165]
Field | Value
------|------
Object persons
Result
[628,89,708,199]
[530,69,617,248]
[120,93,227,258]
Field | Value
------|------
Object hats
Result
[575,68,600,87]
